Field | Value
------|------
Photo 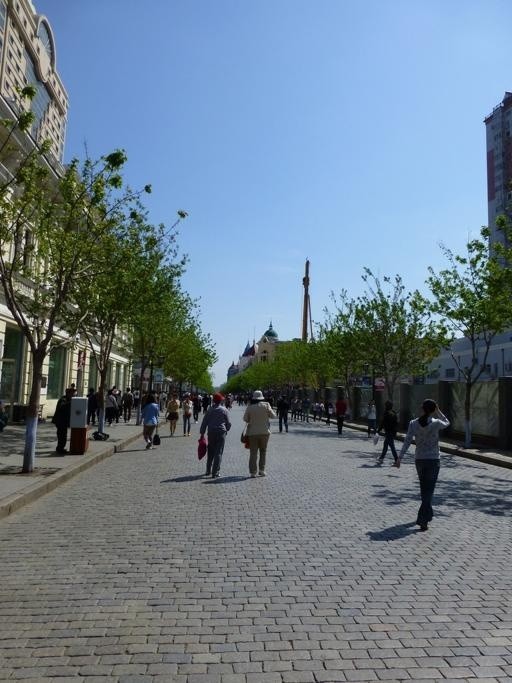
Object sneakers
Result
[194,419,199,424]
[212,472,221,478]
[87,416,131,427]
[184,432,186,436]
[146,440,153,449]
[259,470,266,476]
[417,516,428,531]
[206,470,211,476]
[188,432,193,436]
[392,461,400,466]
[375,458,384,466]
[250,472,257,479]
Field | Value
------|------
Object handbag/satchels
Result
[197,436,207,460]
[373,432,380,444]
[112,388,120,395]
[153,434,160,445]
[241,433,248,443]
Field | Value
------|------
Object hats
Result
[213,393,227,402]
[252,390,266,401]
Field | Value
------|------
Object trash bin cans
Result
[38,404,44,420]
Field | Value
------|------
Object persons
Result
[242,389,277,477]
[374,399,399,466]
[197,392,232,479]
[51,383,348,456]
[395,398,450,529]
[366,398,377,438]
